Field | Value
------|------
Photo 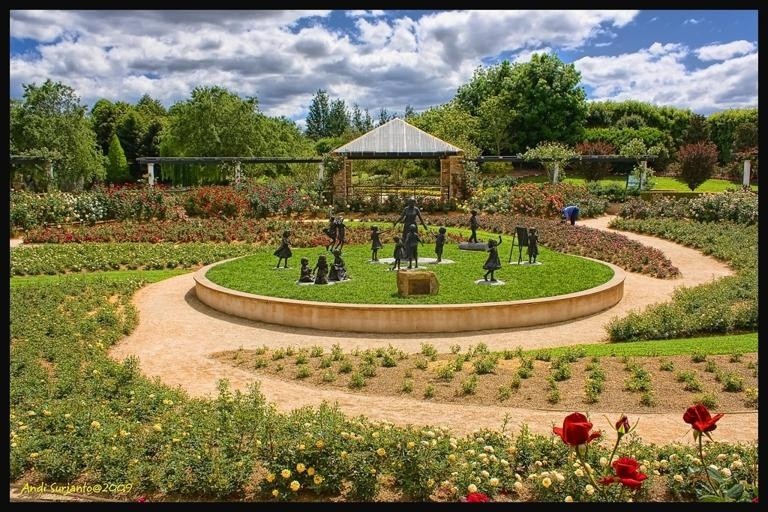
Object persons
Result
[371,225,383,261]
[483,235,502,282]
[561,206,579,226]
[527,227,539,264]
[391,197,430,270]
[299,216,351,285]
[273,230,293,268]
[431,227,446,263]
[468,209,484,243]
